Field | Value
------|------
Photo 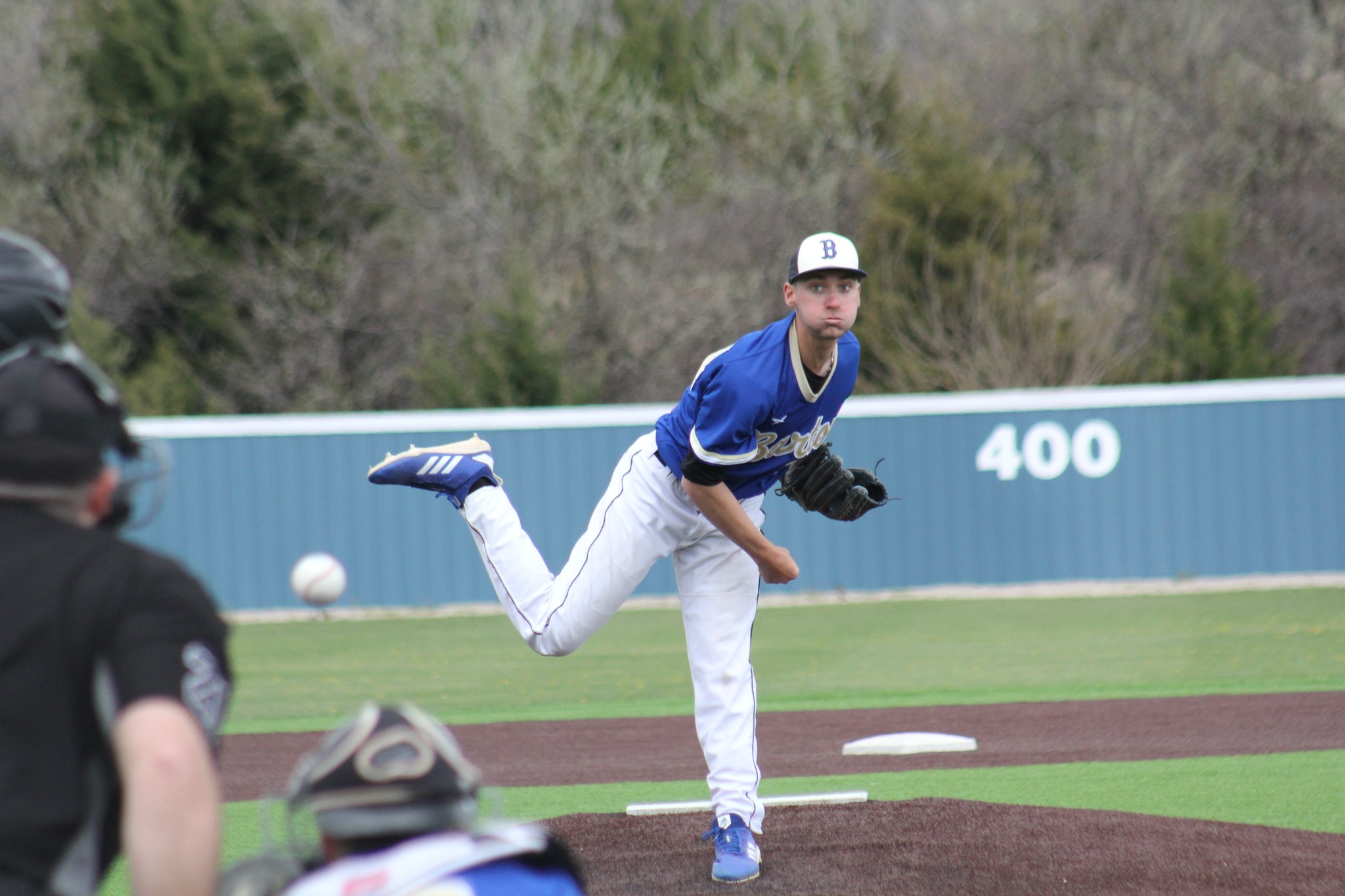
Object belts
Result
[655,449,669,468]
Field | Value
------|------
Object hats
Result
[788,232,870,281]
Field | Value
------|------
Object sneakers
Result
[695,814,760,883]
[368,431,503,509]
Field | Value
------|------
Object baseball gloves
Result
[774,442,903,523]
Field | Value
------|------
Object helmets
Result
[0,346,170,533]
[0,224,75,336]
[274,703,488,846]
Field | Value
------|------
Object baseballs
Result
[288,551,348,611]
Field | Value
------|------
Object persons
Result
[269,698,584,896]
[365,230,863,886]
[0,231,234,894]
